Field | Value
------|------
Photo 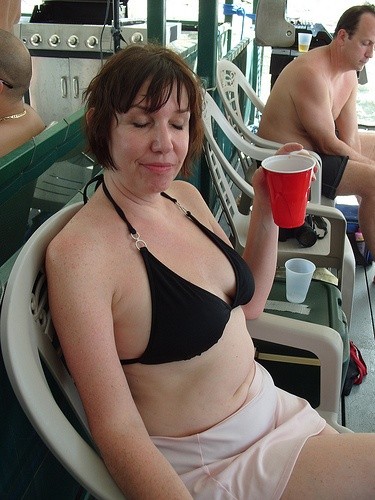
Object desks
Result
[254,271,350,418]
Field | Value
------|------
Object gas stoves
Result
[19,0,181,53]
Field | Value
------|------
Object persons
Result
[0,27,45,155]
[44,45,375,500]
[255,4,375,263]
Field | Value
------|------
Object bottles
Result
[355,228,365,257]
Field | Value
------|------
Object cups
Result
[261,154,316,228]
[298,33,312,52]
[285,258,316,303]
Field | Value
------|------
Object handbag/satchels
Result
[341,339,368,397]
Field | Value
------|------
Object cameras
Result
[278,213,318,247]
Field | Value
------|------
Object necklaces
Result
[0,109,27,122]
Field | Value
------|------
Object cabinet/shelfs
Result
[13,18,186,127]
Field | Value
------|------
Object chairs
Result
[0,202,353,500]
[194,88,359,326]
[216,57,339,205]
